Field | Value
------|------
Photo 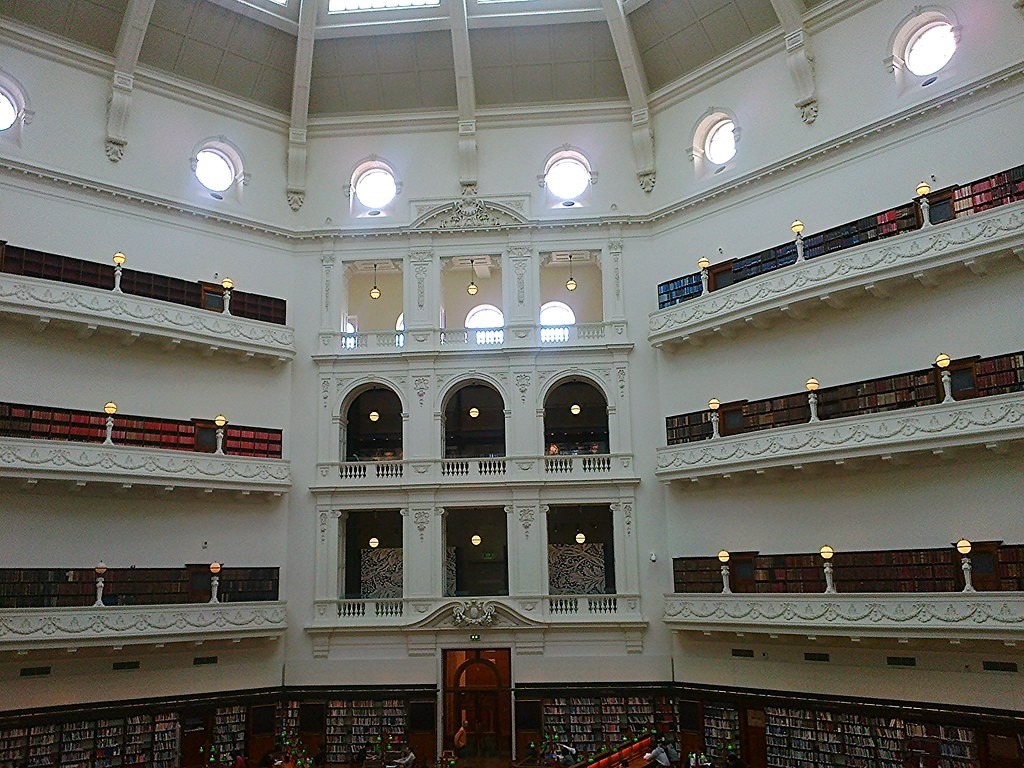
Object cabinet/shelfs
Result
[5,245,286,325]
[538,694,982,767]
[0,699,412,768]
[0,566,280,607]
[666,351,1024,446]
[672,544,1024,592]
[657,164,1024,310]
[0,401,284,460]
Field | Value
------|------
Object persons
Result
[561,748,574,766]
[357,746,373,766]
[393,746,416,768]
[689,746,707,766]
[647,741,678,768]
[232,753,244,768]
[727,753,746,768]
[454,721,469,768]
[315,744,327,766]
[260,751,276,768]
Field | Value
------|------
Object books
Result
[0,569,279,608]
[0,405,281,458]
[668,355,1024,444]
[766,707,979,768]
[0,713,179,768]
[658,165,1024,308]
[276,701,301,758]
[327,700,404,761]
[704,710,740,760]
[210,705,245,768]
[674,547,1024,593]
[542,697,681,759]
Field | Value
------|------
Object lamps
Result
[957,537,972,554]
[916,180,930,195]
[719,549,730,564]
[935,353,951,368]
[95,560,106,575]
[369,263,381,299]
[104,401,117,414]
[806,376,819,390]
[214,414,228,427]
[791,220,804,233]
[819,545,834,559]
[566,255,577,291]
[222,277,233,288]
[467,259,479,296]
[112,251,126,266]
[698,256,709,269]
[708,397,720,410]
[209,561,221,574]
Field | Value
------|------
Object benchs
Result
[442,743,459,762]
